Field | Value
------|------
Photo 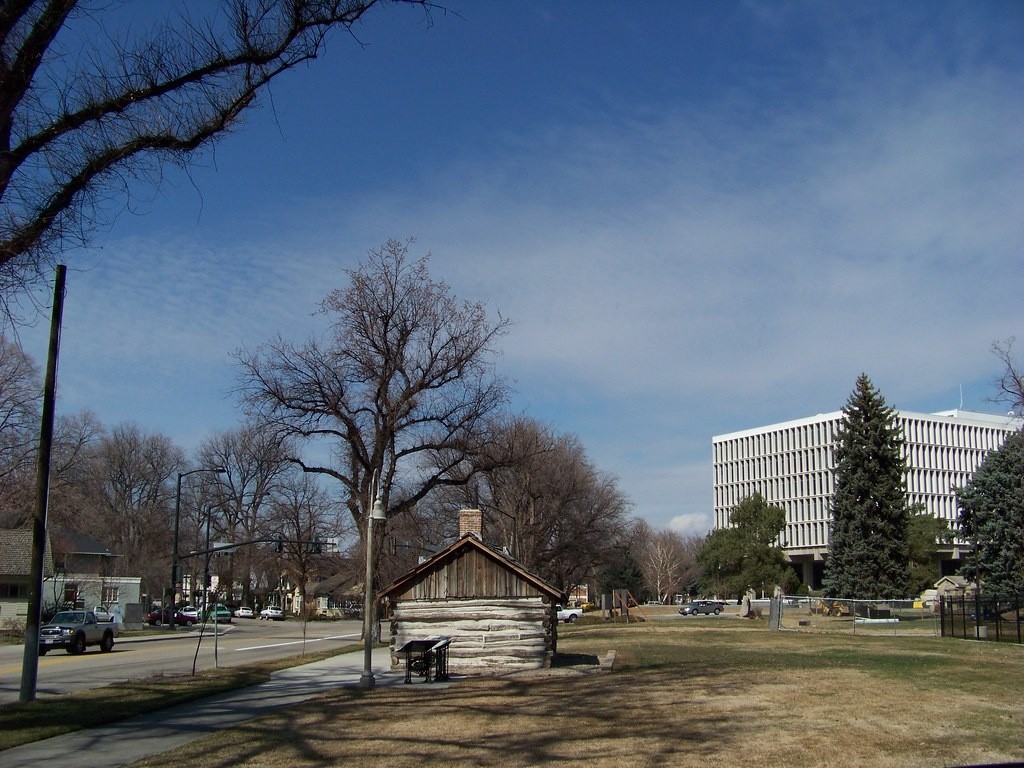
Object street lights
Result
[168,467,227,629]
[360,467,386,688]
[202,491,271,622]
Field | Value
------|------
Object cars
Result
[260,605,285,620]
[678,600,724,616]
[581,601,595,611]
[178,606,199,621]
[198,602,233,622]
[234,606,254,618]
[145,608,197,627]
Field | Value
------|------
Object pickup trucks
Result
[39,609,119,656]
[76,605,116,623]
[555,605,583,623]
